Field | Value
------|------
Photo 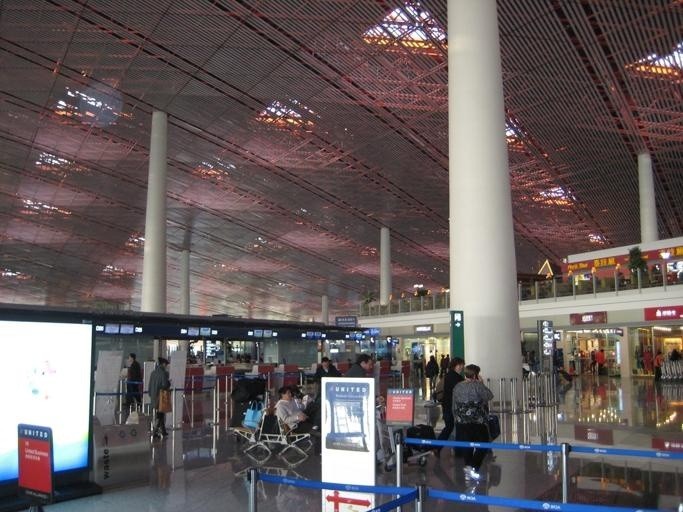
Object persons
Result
[413,353,494,480]
[312,357,340,430]
[591,349,604,375]
[124,353,141,405]
[275,383,320,437]
[553,347,572,381]
[653,349,663,382]
[567,350,577,375]
[149,356,171,438]
[572,347,585,357]
[345,354,373,377]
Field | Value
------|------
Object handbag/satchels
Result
[158,389,172,413]
[407,424,436,453]
[244,400,260,429]
[488,415,501,442]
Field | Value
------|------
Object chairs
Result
[229,408,317,457]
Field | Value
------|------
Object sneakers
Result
[433,447,441,458]
[462,466,480,481]
[152,432,168,438]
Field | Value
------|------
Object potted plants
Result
[626,245,650,288]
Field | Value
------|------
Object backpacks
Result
[435,373,452,404]
